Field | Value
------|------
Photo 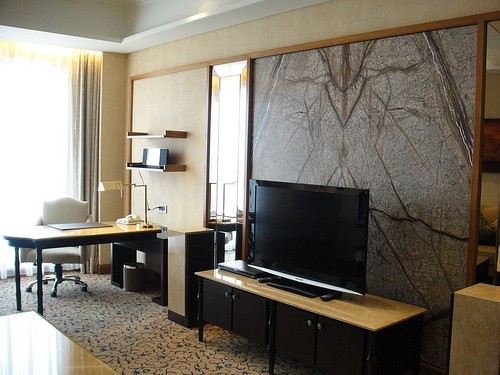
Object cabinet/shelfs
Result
[166,224,213,329]
[449,283,500,375]
[126,130,188,174]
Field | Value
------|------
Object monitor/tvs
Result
[245,180,369,301]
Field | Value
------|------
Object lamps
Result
[98,181,153,228]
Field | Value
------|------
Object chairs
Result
[19,197,89,296]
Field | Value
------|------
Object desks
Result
[3,223,167,315]
[0,310,119,375]
[194,269,428,375]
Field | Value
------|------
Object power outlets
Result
[157,204,167,213]
